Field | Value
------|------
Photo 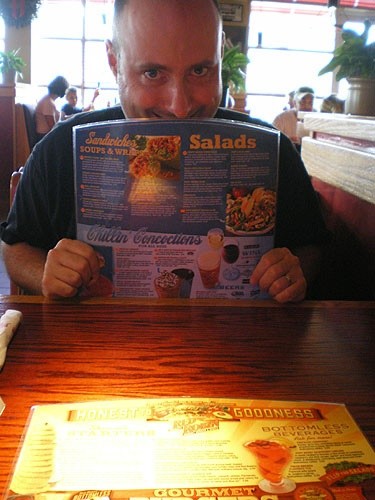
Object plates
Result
[226,221,274,236]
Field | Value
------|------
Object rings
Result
[285,275,293,287]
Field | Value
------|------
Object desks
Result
[0,295,375,500]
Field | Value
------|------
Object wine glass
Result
[221,238,241,280]
[243,436,300,494]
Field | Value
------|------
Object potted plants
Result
[319,28,375,117]
[219,42,249,108]
[0,47,25,85]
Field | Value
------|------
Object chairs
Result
[12,102,43,170]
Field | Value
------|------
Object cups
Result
[207,228,225,252]
[171,268,195,298]
[197,250,222,289]
[153,274,181,298]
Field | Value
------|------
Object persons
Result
[34,75,100,142]
[0,0,335,304]
[273,87,346,145]
